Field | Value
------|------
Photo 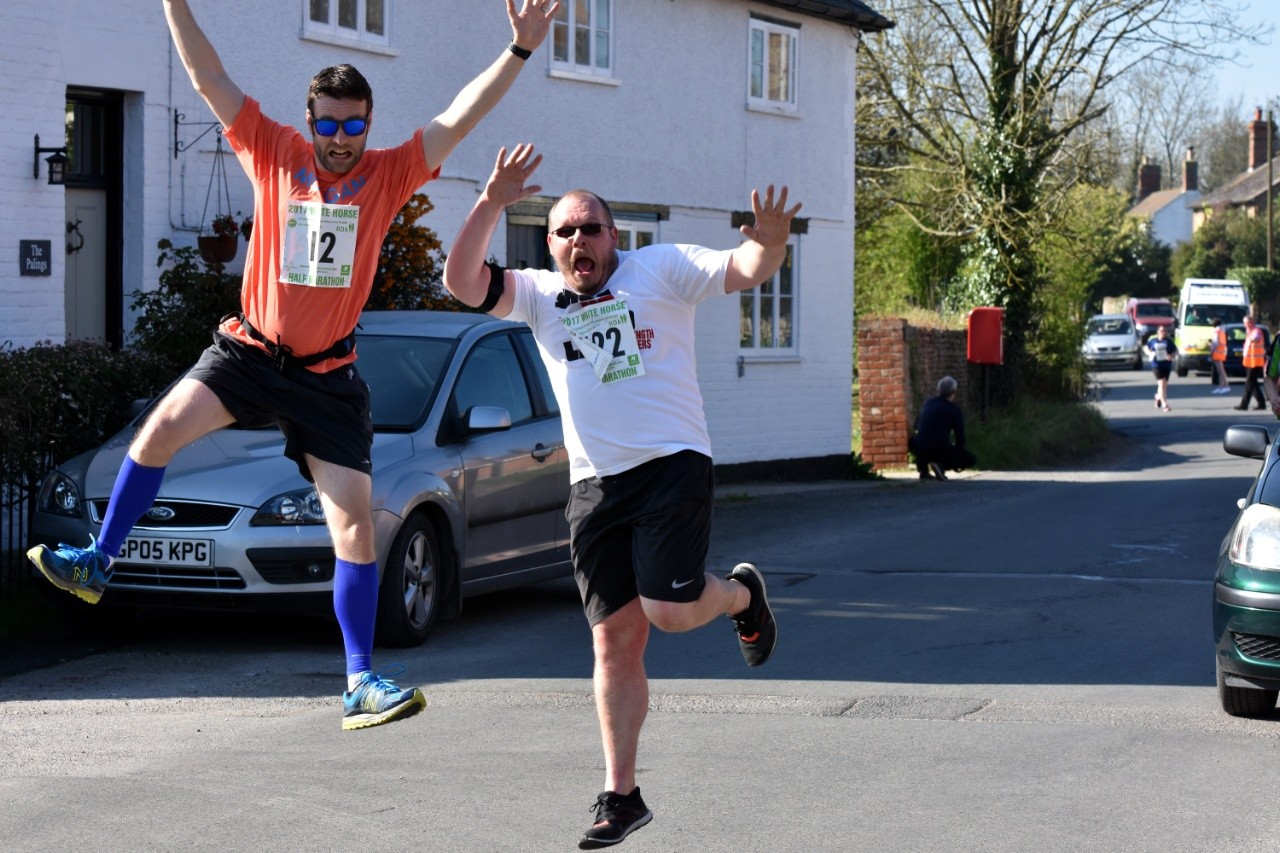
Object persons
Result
[906,375,977,483]
[27,0,562,728]
[1143,314,1280,422]
[443,144,802,846]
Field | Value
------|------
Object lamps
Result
[33,133,68,186]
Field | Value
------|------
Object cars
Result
[32,309,574,648]
[1210,425,1280,719]
[1209,323,1271,385]
[1080,313,1144,371]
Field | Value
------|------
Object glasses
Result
[309,112,369,136]
[549,224,614,238]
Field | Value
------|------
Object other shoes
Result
[1165,406,1171,412]
[1155,394,1161,409]
[1234,405,1248,410]
[919,472,936,481]
[1252,405,1266,410]
[1212,386,1231,394]
[931,463,947,482]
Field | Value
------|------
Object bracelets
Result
[508,38,533,59]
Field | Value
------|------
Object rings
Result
[541,7,546,13]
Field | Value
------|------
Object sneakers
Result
[725,562,778,667]
[26,531,116,604]
[341,670,426,730]
[578,785,653,850]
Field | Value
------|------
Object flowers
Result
[211,211,255,243]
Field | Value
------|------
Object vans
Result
[1172,278,1253,377]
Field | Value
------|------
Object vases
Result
[197,235,238,263]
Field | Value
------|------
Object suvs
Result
[1125,297,1176,345]
